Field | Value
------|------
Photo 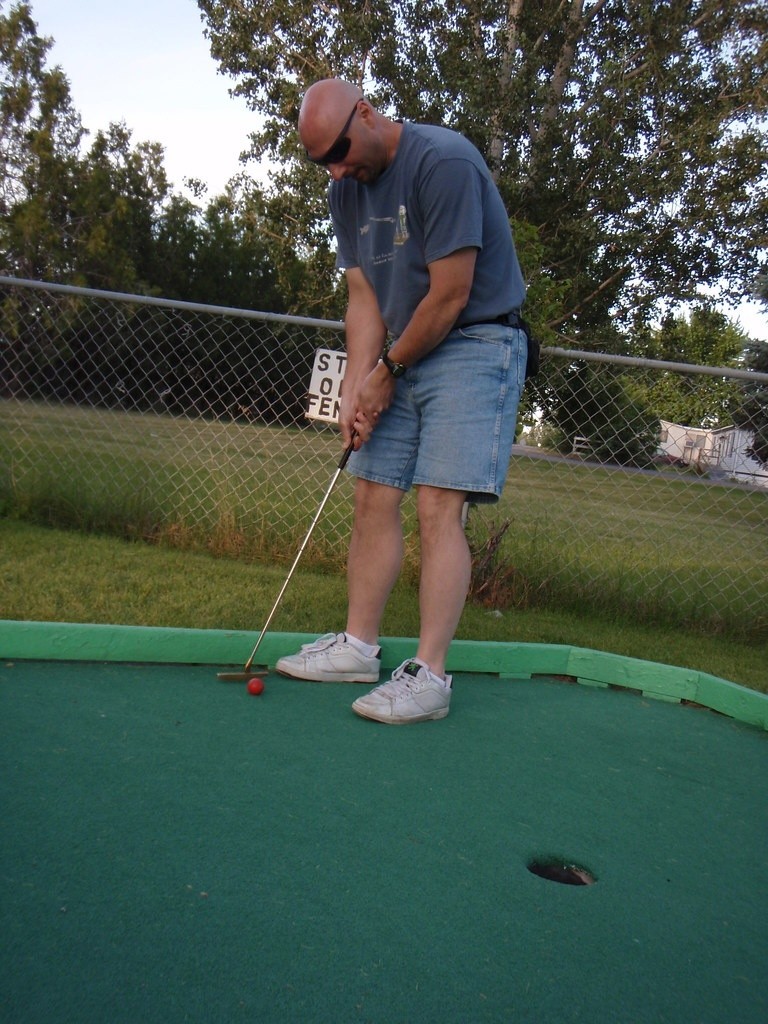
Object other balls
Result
[248,677,264,695]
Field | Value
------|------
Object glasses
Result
[306,98,364,166]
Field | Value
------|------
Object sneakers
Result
[275,632,382,683]
[351,660,451,725]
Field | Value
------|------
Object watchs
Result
[381,348,406,377]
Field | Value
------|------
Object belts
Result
[448,313,531,341]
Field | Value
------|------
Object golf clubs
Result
[217,431,358,680]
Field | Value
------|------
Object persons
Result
[271,77,537,727]
